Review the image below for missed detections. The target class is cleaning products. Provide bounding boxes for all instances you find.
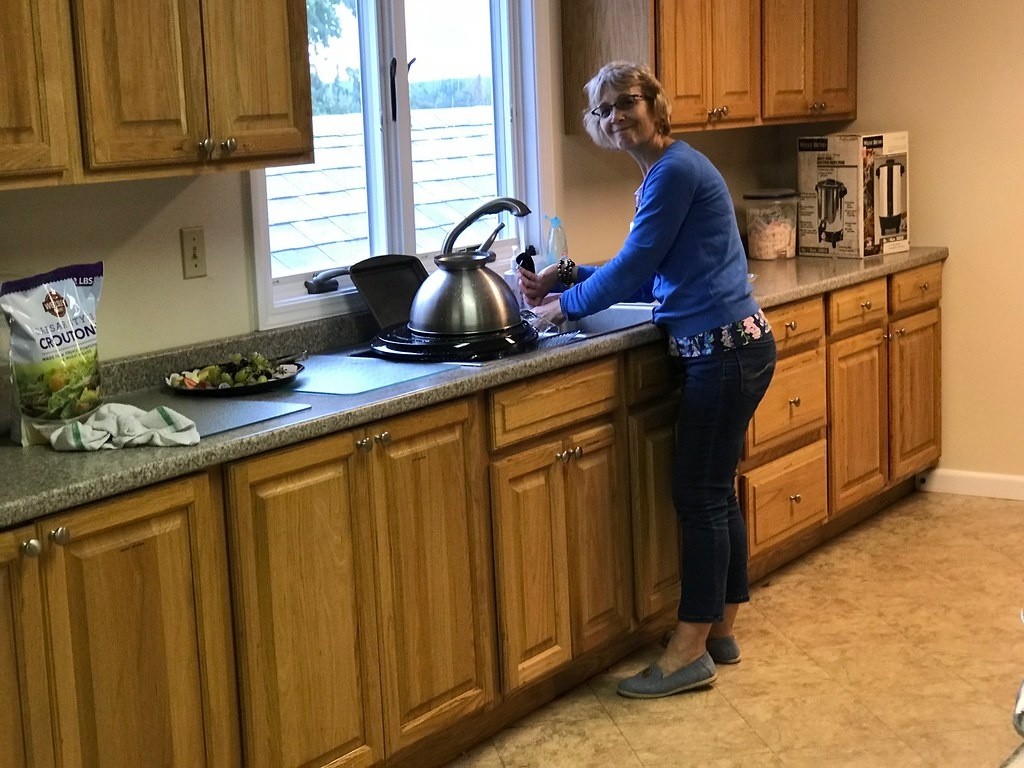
[547,216,570,269]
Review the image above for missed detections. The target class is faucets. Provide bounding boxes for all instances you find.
[443,196,532,254]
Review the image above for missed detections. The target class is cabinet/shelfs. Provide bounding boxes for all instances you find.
[624,338,683,649]
[829,260,942,513]
[560,0,761,136]
[0,0,77,191]
[738,294,831,590]
[482,352,639,732]
[0,464,242,768]
[65,1,316,184]
[213,392,499,768]
[760,0,858,126]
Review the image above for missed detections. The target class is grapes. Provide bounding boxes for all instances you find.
[204,351,275,387]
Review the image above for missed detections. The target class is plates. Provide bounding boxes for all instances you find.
[164,362,305,396]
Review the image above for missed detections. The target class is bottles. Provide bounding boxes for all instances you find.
[545,216,568,265]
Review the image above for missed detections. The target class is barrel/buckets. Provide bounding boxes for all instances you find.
[743,189,800,260]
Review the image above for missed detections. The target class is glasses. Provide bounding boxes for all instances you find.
[591,94,651,118]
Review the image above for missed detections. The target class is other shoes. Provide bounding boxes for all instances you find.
[618,651,719,698]
[663,631,741,663]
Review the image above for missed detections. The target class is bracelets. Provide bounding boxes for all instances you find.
[558,258,576,286]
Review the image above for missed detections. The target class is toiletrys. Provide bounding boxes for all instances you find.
[503,244,521,297]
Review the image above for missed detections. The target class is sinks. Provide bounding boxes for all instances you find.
[517,293,659,338]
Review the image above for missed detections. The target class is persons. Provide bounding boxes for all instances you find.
[518,59,778,700]
